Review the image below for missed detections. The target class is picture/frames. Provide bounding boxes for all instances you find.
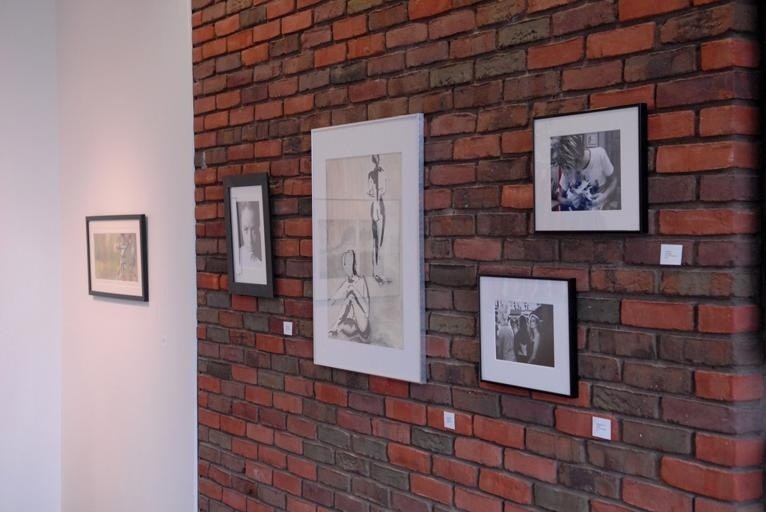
[84,213,148,303]
[533,101,649,236]
[311,111,426,388]
[222,171,274,299]
[481,273,580,398]
